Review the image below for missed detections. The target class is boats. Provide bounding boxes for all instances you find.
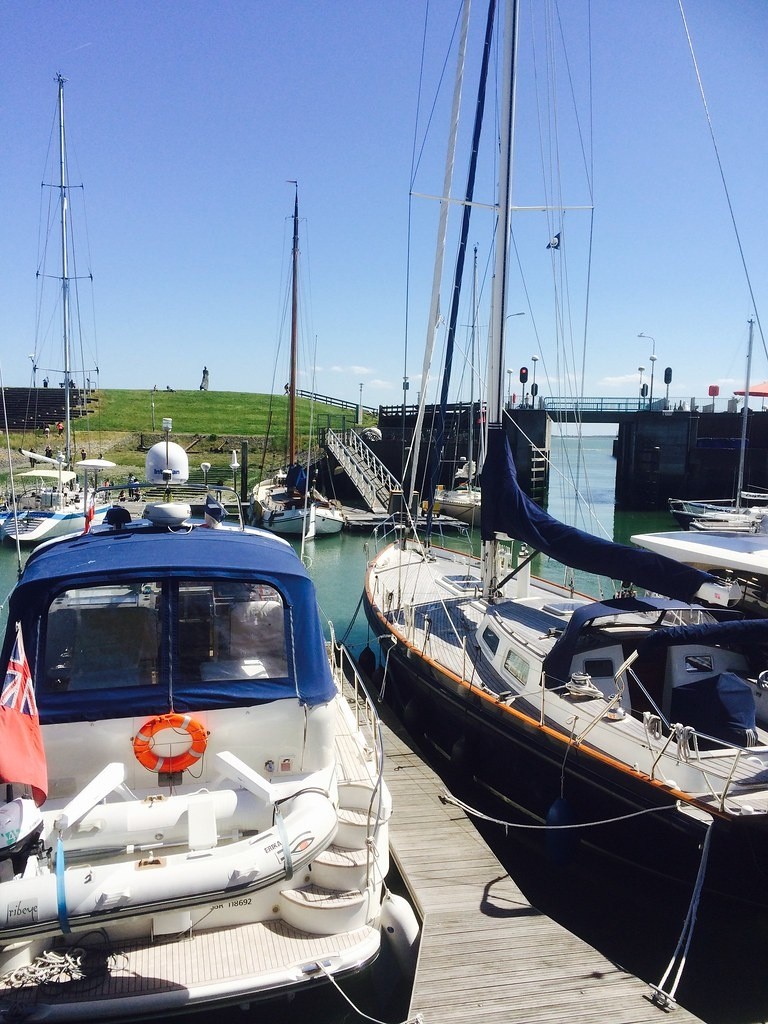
[0,468,423,1024]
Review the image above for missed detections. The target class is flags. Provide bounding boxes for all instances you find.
[81,498,94,535]
[200,495,227,529]
[0,622,47,807]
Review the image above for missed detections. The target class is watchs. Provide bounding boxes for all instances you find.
[57,422,64,435]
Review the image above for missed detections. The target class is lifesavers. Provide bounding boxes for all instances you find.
[132,713,208,773]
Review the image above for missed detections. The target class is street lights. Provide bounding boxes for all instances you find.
[358,383,365,424]
[506,369,514,408]
[635,332,657,411]
[403,377,408,407]
[530,356,539,408]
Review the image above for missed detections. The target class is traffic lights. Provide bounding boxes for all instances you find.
[520,367,527,383]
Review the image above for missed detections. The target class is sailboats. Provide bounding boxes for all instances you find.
[0,70,346,544]
[351,0,767,897]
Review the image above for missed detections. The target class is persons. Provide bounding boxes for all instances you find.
[29,447,35,467]
[45,428,49,438]
[283,383,288,395]
[98,454,104,472]
[31,492,35,497]
[118,490,126,502]
[81,450,87,460]
[127,473,142,502]
[103,479,109,503]
[45,446,53,458]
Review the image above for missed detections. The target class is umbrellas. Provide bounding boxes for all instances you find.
[733,382,768,397]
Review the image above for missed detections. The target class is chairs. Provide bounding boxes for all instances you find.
[202,600,288,681]
[67,607,159,691]
